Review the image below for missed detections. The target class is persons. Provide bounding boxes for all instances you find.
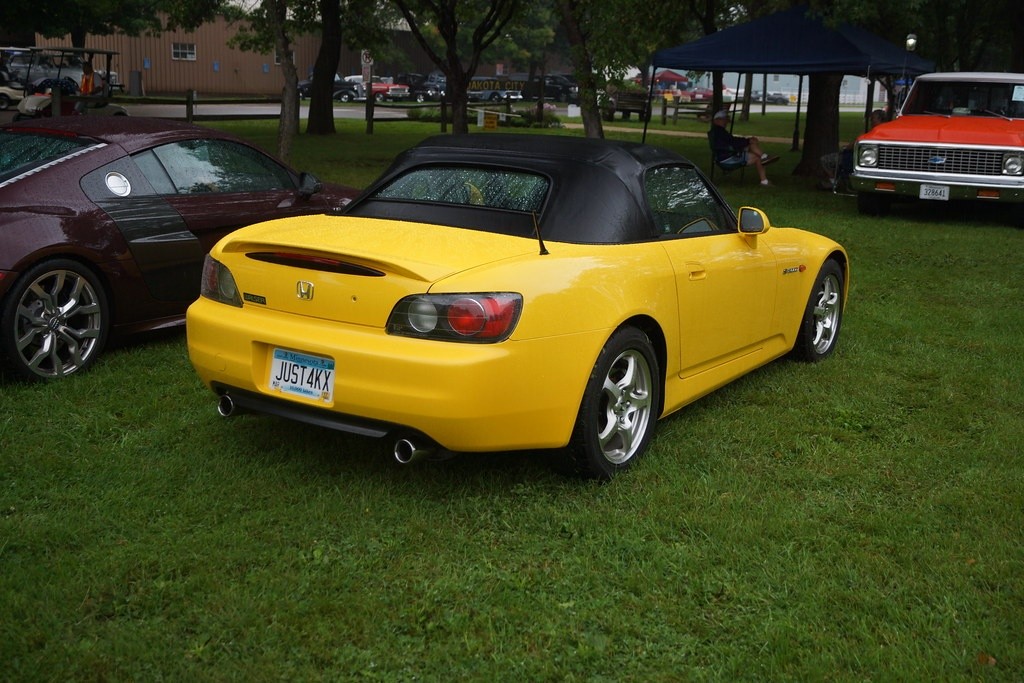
[819,108,885,188]
[710,108,776,187]
[81,61,103,96]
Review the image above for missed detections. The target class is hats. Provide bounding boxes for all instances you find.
[713,110,729,119]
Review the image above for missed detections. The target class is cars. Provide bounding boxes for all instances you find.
[0,114,365,385]
[531,74,580,100]
[0,46,122,111]
[298,72,524,104]
[849,71,1024,218]
[682,87,790,105]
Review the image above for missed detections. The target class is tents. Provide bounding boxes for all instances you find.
[638,6,938,148]
[647,70,688,89]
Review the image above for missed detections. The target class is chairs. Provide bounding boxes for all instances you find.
[707,128,768,188]
[835,140,860,193]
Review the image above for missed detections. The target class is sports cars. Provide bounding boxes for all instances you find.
[186,132,850,478]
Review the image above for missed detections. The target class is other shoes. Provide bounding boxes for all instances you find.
[759,180,776,188]
[761,155,780,166]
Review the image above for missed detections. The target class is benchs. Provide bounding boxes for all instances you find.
[696,102,732,125]
[600,92,656,122]
